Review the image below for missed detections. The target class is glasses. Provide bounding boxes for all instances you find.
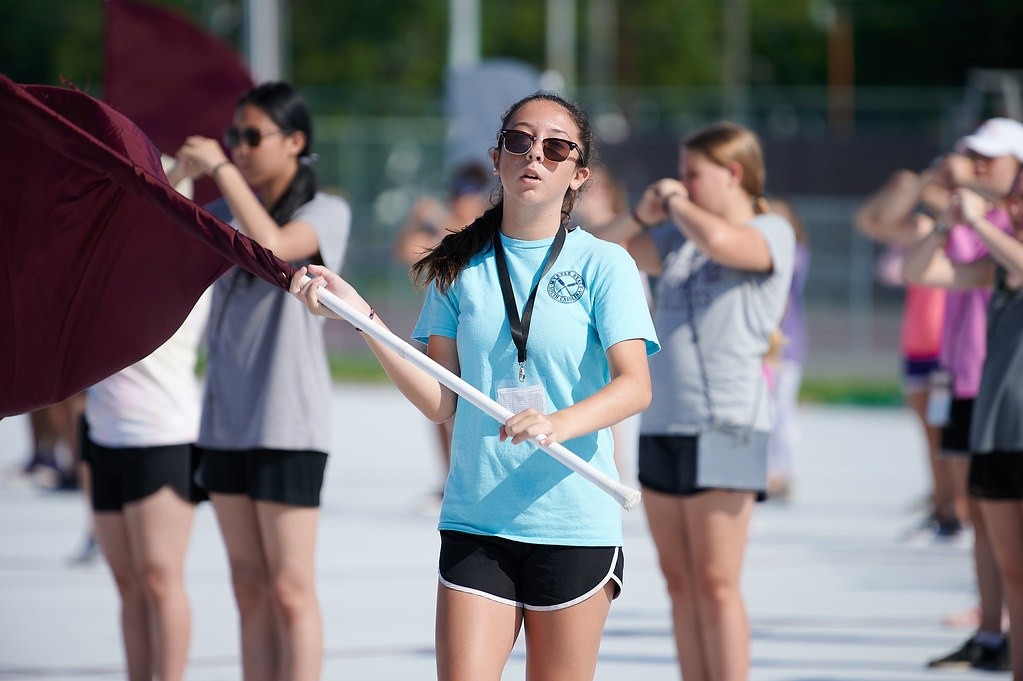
[223,126,290,149]
[495,131,586,167]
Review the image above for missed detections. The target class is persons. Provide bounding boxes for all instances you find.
[289,90,660,680]
[579,164,657,275]
[25,392,87,491]
[165,78,350,680]
[82,153,214,679]
[757,202,811,498]
[597,121,796,678]
[856,120,1022,680]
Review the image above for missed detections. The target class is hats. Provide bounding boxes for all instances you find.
[957,118,1023,162]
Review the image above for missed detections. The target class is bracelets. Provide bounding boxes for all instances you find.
[932,221,951,237]
[354,306,374,331]
[661,191,689,219]
[630,206,655,232]
[211,161,234,182]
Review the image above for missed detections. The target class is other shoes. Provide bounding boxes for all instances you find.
[928,632,1009,676]
[913,513,970,547]
